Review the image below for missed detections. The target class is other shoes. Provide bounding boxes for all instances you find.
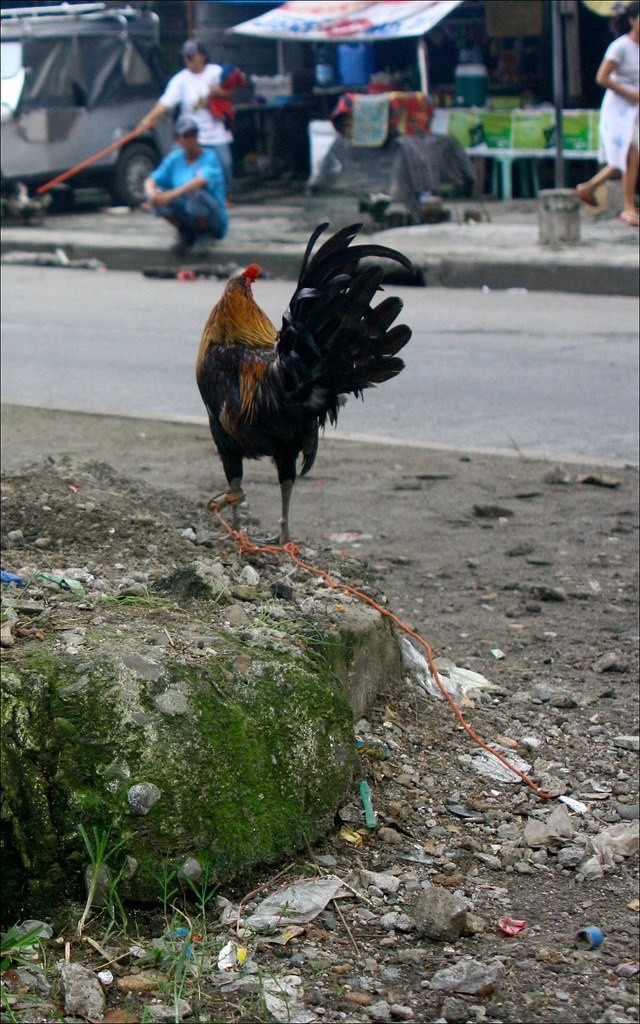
[170,232,196,255]
[576,183,599,206]
[192,236,215,256]
[620,210,640,225]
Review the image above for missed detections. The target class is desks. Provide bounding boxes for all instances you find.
[314,86,369,119]
[228,103,303,178]
[467,145,601,158]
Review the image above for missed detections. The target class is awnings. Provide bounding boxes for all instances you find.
[222,1,468,92]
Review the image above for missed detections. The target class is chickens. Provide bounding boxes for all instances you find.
[194,223,417,526]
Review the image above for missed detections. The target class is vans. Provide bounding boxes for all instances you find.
[1,0,174,208]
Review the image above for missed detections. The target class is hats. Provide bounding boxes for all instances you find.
[180,39,205,57]
[175,117,199,135]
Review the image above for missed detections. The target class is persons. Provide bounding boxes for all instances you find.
[575,2,639,227]
[142,114,228,259]
[136,39,235,203]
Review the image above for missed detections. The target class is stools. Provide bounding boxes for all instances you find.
[494,153,541,199]
